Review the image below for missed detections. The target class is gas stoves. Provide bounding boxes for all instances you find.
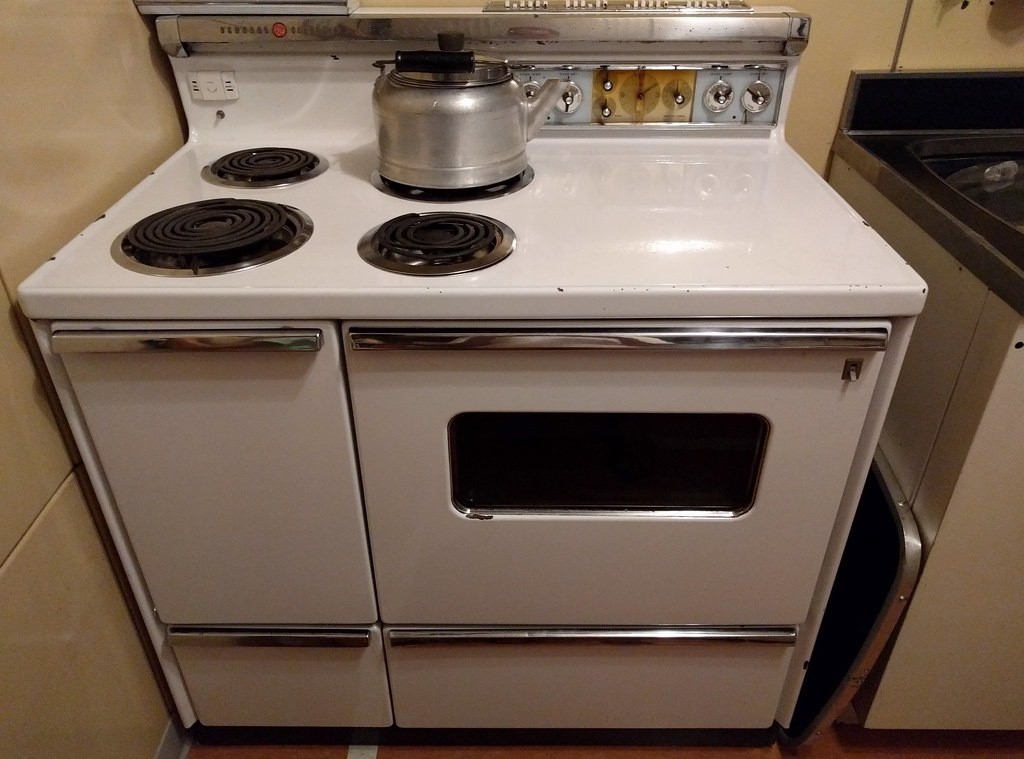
[15,6,929,317]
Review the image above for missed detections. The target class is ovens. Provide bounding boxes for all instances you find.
[30,319,900,730]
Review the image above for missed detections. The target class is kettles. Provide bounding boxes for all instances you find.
[371,32,571,202]
